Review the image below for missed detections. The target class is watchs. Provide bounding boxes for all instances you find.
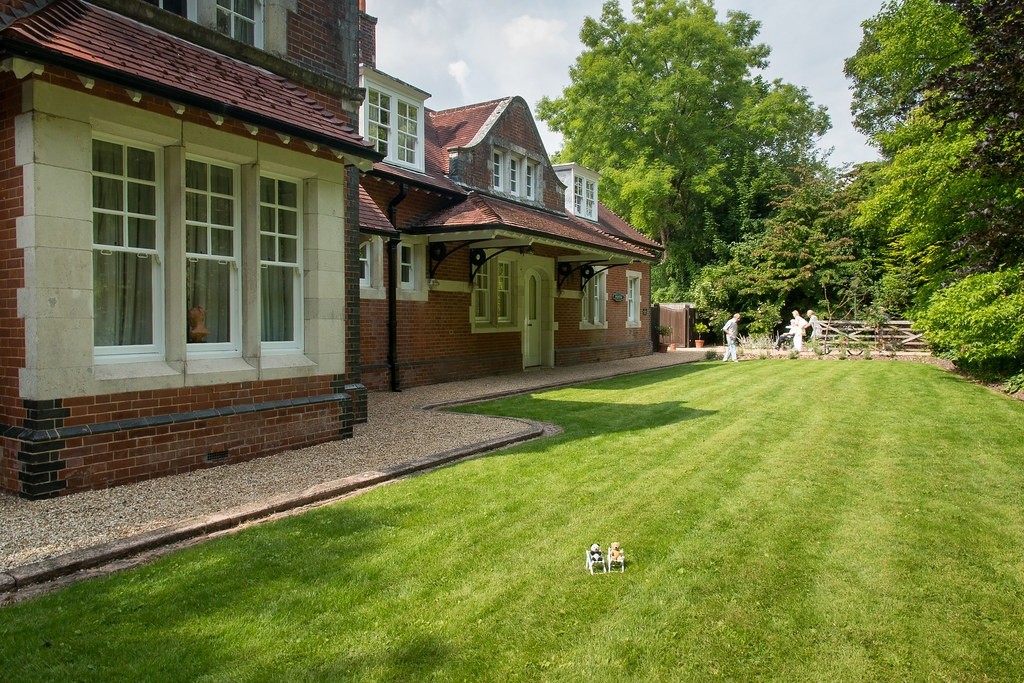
[722,313,741,362]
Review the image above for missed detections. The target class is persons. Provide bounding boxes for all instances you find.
[774,319,795,349]
[804,310,822,351]
[791,310,808,352]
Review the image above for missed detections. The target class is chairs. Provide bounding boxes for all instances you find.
[607,547,625,574]
[585,549,607,575]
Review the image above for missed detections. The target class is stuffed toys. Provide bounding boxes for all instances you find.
[591,542,624,560]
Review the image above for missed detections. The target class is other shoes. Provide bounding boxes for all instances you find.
[734,359,738,362]
[722,358,729,362]
[773,345,780,349]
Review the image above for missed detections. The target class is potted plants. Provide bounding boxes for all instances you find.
[655,324,673,353]
[693,322,710,348]
